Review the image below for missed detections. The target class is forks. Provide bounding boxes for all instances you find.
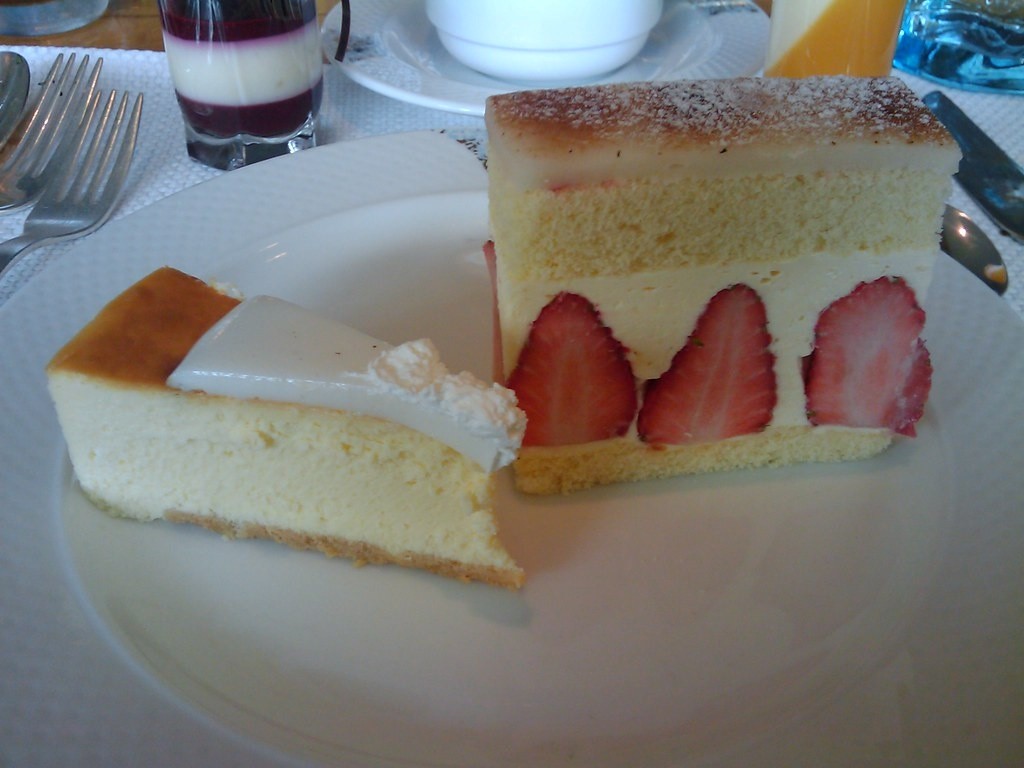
[0,89,143,273]
[0,53,103,218]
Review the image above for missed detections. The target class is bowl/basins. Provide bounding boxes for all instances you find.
[425,0,665,82]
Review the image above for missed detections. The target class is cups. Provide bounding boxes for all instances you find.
[763,0,905,79]
[0,0,110,37]
[894,0,1024,96]
[154,0,323,168]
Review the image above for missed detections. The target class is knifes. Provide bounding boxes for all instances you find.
[919,91,1024,239]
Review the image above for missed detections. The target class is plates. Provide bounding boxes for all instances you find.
[0,132,1024,768]
[316,0,773,117]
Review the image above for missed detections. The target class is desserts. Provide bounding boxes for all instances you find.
[480,79,959,495]
[43,266,526,588]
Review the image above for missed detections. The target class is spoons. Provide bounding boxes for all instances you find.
[938,205,1009,295]
[0,51,31,154]
